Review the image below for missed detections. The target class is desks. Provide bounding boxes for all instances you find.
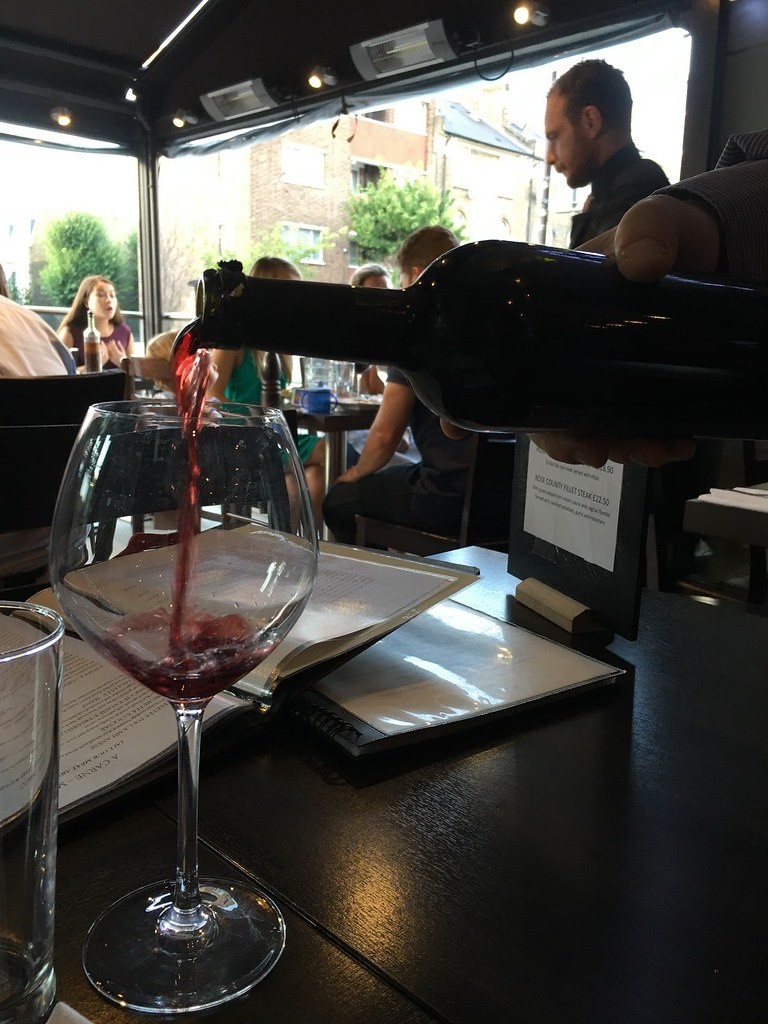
[683,481,768,606]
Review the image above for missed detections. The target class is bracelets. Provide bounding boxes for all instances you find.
[352,465,368,478]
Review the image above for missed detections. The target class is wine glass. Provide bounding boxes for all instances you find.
[48,398,320,1014]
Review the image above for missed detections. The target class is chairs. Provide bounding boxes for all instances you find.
[0,358,132,613]
[0,546,768,1024]
[354,432,515,553]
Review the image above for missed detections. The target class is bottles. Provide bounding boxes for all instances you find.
[82,310,103,374]
[194,242,768,487]
[259,352,282,408]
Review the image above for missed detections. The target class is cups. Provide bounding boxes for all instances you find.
[301,381,338,415]
[0,600,66,1024]
[300,357,356,399]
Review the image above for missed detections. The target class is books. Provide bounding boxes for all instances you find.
[0,526,482,828]
[285,597,629,756]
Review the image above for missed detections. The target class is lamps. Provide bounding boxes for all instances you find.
[513,4,550,28]
[172,108,199,128]
[307,64,338,90]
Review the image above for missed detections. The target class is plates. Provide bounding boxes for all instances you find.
[331,396,383,413]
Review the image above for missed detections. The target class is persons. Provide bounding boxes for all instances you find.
[56,274,136,375]
[202,257,327,536]
[544,60,671,250]
[144,332,182,400]
[322,227,510,547]
[352,264,393,395]
[440,127,768,468]
[0,262,78,379]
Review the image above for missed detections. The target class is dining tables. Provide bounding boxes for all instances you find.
[282,398,379,540]
